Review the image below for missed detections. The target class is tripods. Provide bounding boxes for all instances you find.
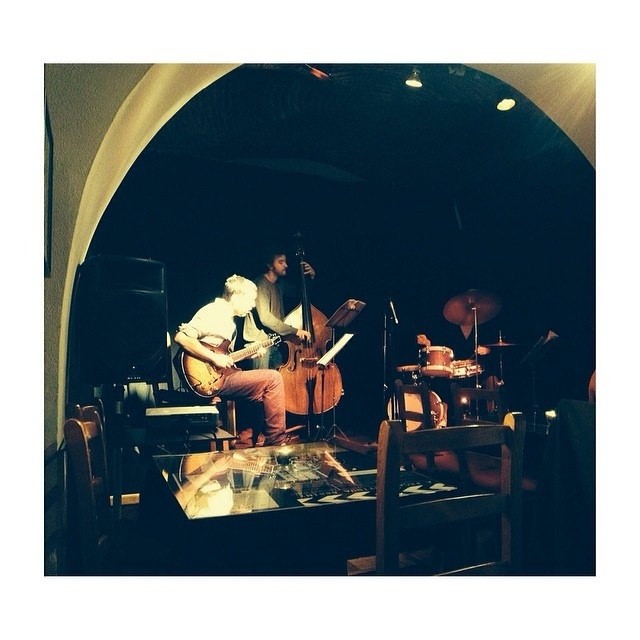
[313,300,365,445]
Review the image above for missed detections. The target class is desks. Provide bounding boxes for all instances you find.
[109,425,236,519]
[153,441,461,575]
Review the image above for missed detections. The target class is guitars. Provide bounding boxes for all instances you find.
[176,333,281,399]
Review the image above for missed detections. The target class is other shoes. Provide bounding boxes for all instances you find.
[234,435,253,449]
[264,433,300,445]
[256,434,266,446]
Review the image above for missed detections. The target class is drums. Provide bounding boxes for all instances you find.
[388,385,443,432]
[418,346,454,377]
[397,365,431,394]
[450,361,483,403]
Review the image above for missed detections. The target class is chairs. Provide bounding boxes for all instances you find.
[64,404,111,576]
[153,333,238,452]
[346,377,537,577]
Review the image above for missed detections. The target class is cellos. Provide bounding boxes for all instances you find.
[278,232,342,439]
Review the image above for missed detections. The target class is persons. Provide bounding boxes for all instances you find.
[174,273,301,446]
[238,247,315,446]
[416,324,492,423]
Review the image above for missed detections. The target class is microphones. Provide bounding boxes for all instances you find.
[389,300,398,324]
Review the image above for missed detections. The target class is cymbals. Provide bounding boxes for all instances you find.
[481,343,520,348]
[443,289,501,326]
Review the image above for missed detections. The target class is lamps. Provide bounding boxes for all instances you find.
[405,65,423,88]
[496,86,516,111]
[305,64,333,80]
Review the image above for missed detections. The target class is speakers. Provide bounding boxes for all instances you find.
[79,256,172,387]
